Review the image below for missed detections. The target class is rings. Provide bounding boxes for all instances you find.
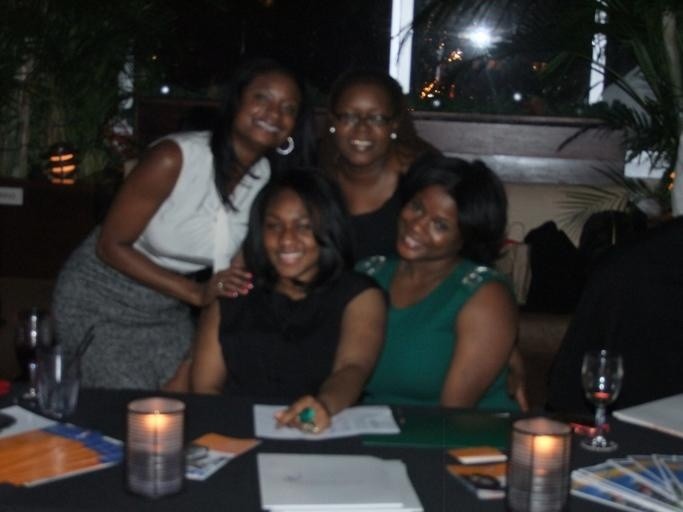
[298,407,313,423]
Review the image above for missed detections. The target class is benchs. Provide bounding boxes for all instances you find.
[491,181,661,408]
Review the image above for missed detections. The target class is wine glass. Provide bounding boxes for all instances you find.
[14,306,55,406]
[580,350,623,453]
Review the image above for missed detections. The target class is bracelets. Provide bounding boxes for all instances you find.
[217,282,224,289]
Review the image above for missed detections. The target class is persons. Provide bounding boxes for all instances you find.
[51,53,303,392]
[192,168,388,434]
[349,158,530,416]
[326,72,515,281]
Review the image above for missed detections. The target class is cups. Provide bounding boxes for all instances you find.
[37,350,79,422]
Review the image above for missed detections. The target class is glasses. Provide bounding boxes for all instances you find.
[340,113,397,127]
